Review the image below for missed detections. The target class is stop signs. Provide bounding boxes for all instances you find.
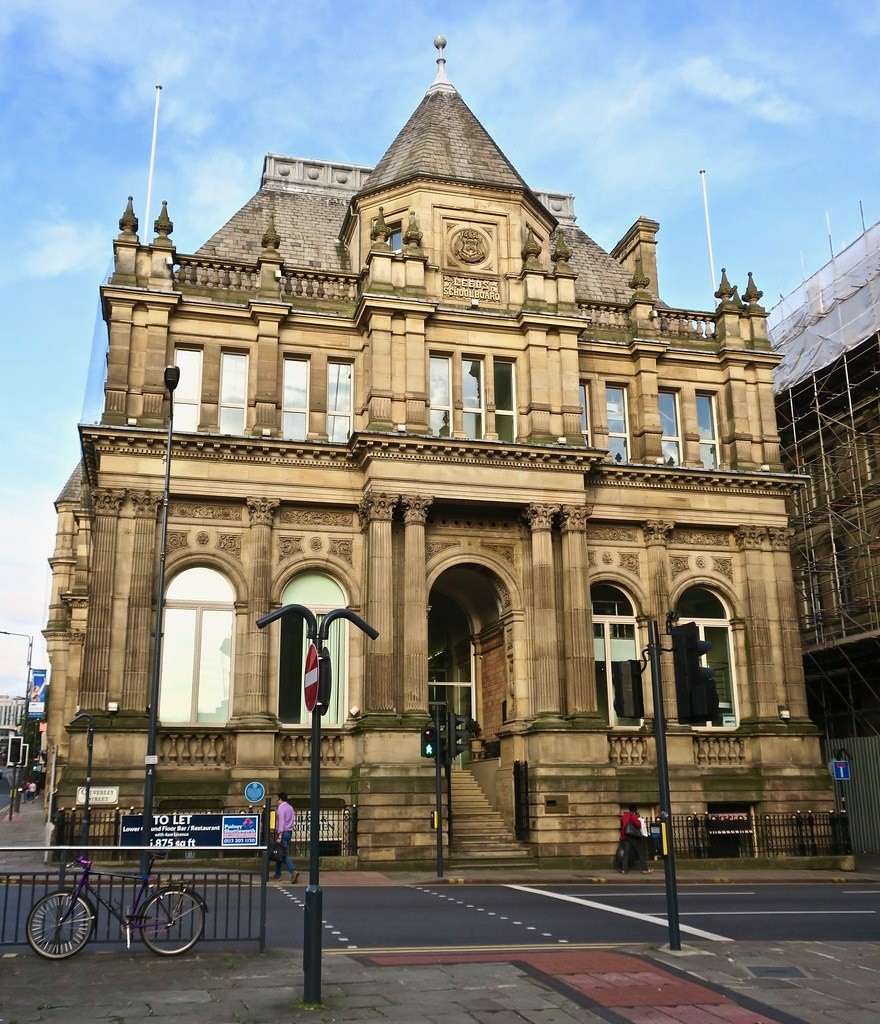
[303,642,320,712]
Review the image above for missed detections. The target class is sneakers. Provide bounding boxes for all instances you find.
[621,869,628,874]
[642,869,654,874]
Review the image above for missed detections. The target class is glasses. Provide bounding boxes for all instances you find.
[277,798,280,801]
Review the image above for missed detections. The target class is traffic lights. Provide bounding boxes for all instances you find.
[671,625,719,723]
[421,726,438,758]
[32,758,39,771]
[449,712,469,759]
[6,736,23,765]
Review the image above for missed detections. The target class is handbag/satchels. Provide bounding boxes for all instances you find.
[616,844,624,865]
[270,843,287,863]
[624,823,642,839]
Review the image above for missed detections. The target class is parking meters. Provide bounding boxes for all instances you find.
[270,811,276,830]
[648,821,667,858]
[431,811,438,829]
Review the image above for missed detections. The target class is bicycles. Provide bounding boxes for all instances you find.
[25,849,210,961]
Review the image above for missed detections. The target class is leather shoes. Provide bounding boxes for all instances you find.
[271,874,281,881]
[291,871,299,885]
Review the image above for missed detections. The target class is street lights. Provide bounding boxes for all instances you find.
[0,632,34,745]
[138,365,181,880]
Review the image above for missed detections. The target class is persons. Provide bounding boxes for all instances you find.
[620,804,655,874]
[20,779,40,804]
[271,793,299,884]
